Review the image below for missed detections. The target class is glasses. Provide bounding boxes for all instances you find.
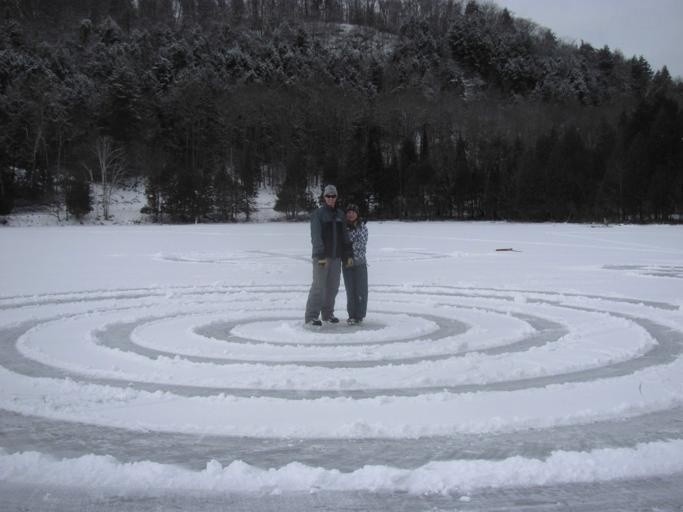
[324,192,337,199]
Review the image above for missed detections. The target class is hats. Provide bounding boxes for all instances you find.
[323,184,338,196]
[345,203,361,215]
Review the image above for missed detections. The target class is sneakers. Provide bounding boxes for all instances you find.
[347,315,365,324]
[303,317,323,327]
[322,313,341,322]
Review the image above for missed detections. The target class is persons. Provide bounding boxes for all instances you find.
[304,184,354,326]
[340,205,368,324]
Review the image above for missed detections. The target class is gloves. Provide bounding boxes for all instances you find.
[343,256,354,268]
[317,255,329,268]
